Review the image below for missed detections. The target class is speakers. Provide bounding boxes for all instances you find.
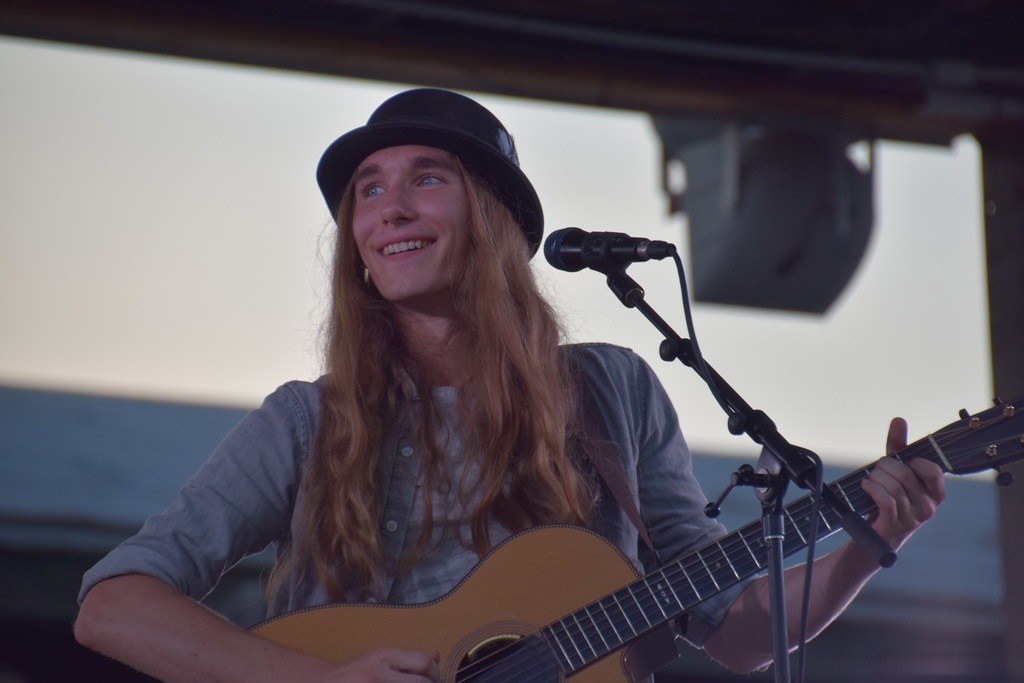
[679,125,872,314]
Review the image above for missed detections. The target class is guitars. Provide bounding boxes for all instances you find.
[247,397,1023,681]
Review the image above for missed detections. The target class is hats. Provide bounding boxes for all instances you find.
[316,87,544,263]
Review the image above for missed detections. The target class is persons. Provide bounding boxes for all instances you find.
[71,89,950,683]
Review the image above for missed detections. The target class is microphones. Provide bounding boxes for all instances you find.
[544,227,676,272]
